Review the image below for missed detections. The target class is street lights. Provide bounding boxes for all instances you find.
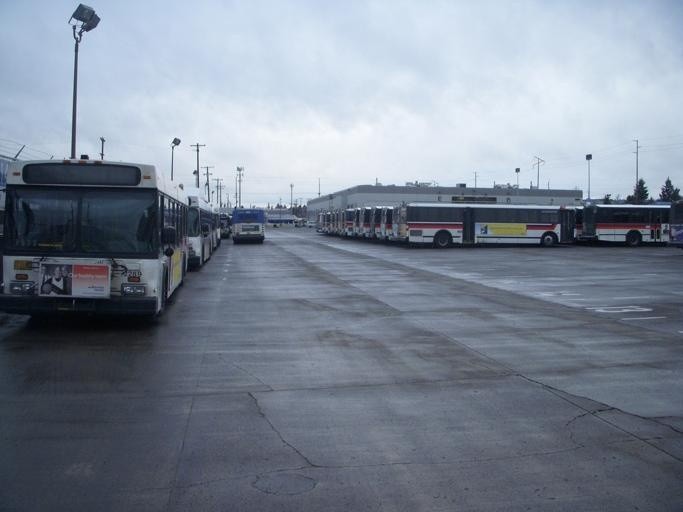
[67,3,100,160]
[170,137,180,180]
[585,154,592,198]
[515,168,519,186]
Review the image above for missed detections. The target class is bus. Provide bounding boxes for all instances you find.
[0,160,231,319]
[267,218,296,228]
[230,207,264,243]
